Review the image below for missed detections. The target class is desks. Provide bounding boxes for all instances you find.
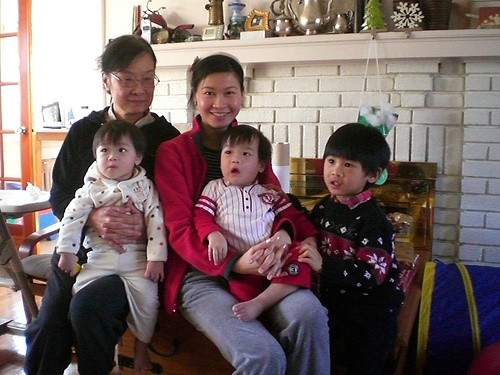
[0,189,52,336]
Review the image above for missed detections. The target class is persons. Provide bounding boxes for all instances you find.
[154,52,330,375]
[193,125,319,321]
[23,35,181,375]
[262,123,404,375]
[56,120,167,373]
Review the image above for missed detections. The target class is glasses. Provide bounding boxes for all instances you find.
[107,73,162,90]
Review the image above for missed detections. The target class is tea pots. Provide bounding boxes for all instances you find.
[288,0,334,36]
[269,0,300,37]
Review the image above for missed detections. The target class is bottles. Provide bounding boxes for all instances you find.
[271,142,290,193]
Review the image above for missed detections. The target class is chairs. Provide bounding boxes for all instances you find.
[19,222,60,325]
[290,158,439,375]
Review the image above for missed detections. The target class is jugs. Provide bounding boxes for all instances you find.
[332,9,354,33]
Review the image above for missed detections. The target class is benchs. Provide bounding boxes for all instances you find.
[118,313,236,375]
